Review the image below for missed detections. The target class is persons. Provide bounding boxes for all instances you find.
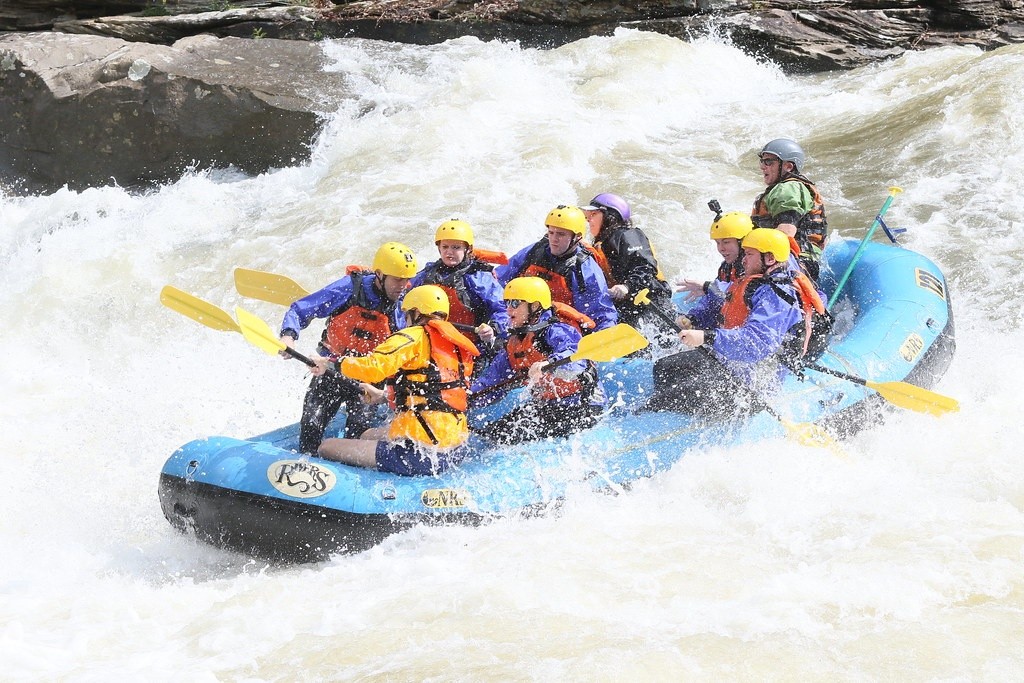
[279,242,417,460]
[307,284,481,477]
[492,205,618,340]
[400,219,509,378]
[466,276,607,444]
[579,192,674,356]
[750,139,828,281]
[636,228,833,417]
[677,212,756,329]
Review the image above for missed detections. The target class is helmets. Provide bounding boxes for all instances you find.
[401,285,449,314]
[741,228,790,262]
[545,205,586,237]
[580,193,630,222]
[504,276,552,310]
[761,138,805,172]
[372,242,417,279]
[435,218,474,245]
[710,211,753,239]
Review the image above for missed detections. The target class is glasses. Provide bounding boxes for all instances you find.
[504,300,519,309]
[760,157,779,166]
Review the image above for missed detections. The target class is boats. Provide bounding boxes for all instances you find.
[156,239,958,571]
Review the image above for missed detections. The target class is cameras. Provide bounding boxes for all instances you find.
[708,200,722,213]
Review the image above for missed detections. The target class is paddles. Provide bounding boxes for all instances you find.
[799,358,960,418]
[634,287,838,446]
[232,265,478,335]
[234,303,369,396]
[828,184,904,311]
[468,324,651,402]
[158,284,243,337]
